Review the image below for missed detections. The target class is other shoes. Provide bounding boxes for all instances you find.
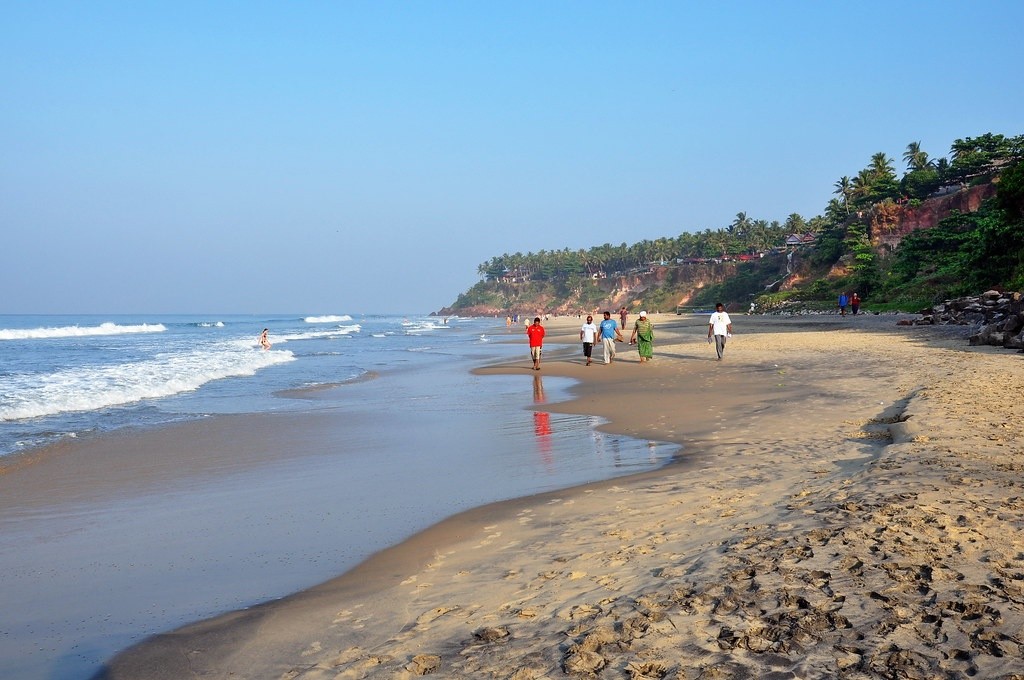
[586,363,590,366]
[536,367,540,370]
[532,367,536,370]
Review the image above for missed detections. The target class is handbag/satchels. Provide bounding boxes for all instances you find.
[848,297,852,306]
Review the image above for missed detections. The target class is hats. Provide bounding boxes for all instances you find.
[639,311,646,317]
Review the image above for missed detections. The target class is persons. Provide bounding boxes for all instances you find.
[838,291,860,317]
[580,316,597,366]
[631,311,655,364]
[597,311,623,365]
[507,314,520,326]
[524,317,531,330]
[620,307,627,330]
[708,303,731,361]
[527,318,545,370]
[258,329,270,350]
